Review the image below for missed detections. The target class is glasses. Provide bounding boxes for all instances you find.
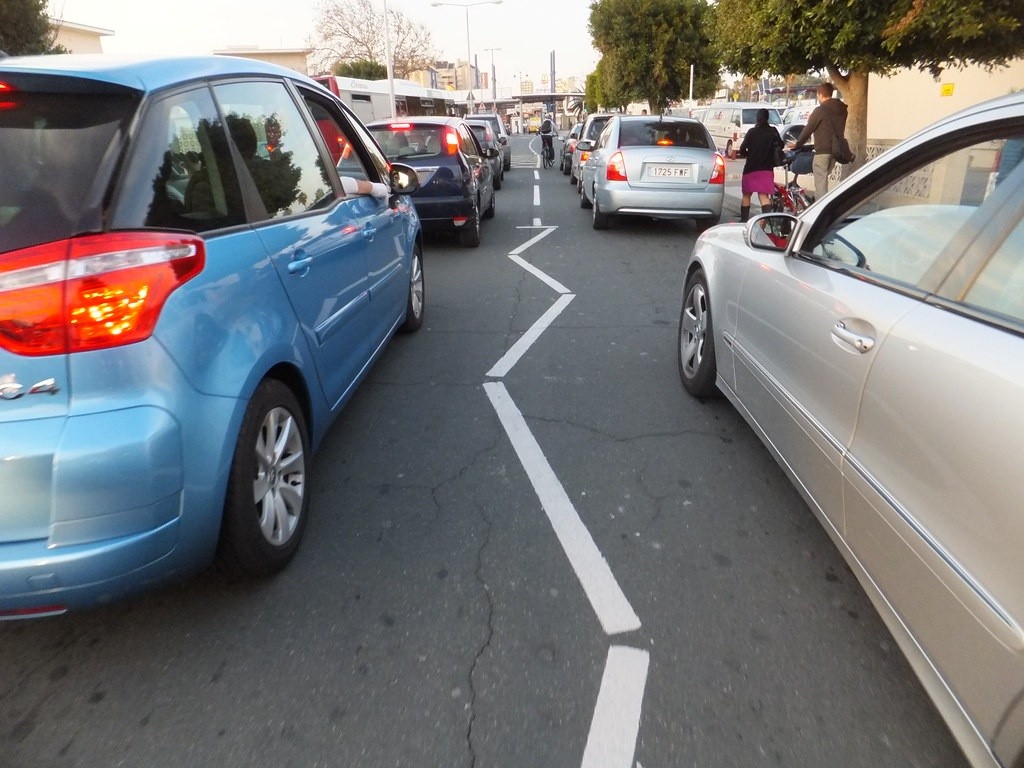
[394,176,400,180]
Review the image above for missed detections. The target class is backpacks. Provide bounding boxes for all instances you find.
[541,119,551,134]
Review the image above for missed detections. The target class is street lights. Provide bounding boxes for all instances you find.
[430,0,504,114]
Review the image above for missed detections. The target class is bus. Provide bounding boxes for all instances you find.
[299,72,460,171]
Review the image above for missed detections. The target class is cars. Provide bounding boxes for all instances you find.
[331,113,514,251]
[559,112,727,233]
[669,86,1024,767]
[1,52,431,633]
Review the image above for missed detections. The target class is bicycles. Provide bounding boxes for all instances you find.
[538,142,555,167]
[766,149,815,216]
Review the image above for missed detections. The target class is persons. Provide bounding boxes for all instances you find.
[739,108,785,224]
[338,176,388,199]
[535,114,560,163]
[641,109,647,116]
[786,82,849,202]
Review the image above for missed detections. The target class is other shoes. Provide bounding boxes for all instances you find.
[541,150,544,155]
[550,160,555,163]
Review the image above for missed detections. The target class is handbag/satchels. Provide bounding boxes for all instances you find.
[771,127,786,166]
[832,135,856,164]
[792,152,813,174]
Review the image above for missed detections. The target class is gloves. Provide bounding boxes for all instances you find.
[369,181,388,206]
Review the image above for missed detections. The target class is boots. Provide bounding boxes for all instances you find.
[761,204,772,213]
[739,204,750,223]
